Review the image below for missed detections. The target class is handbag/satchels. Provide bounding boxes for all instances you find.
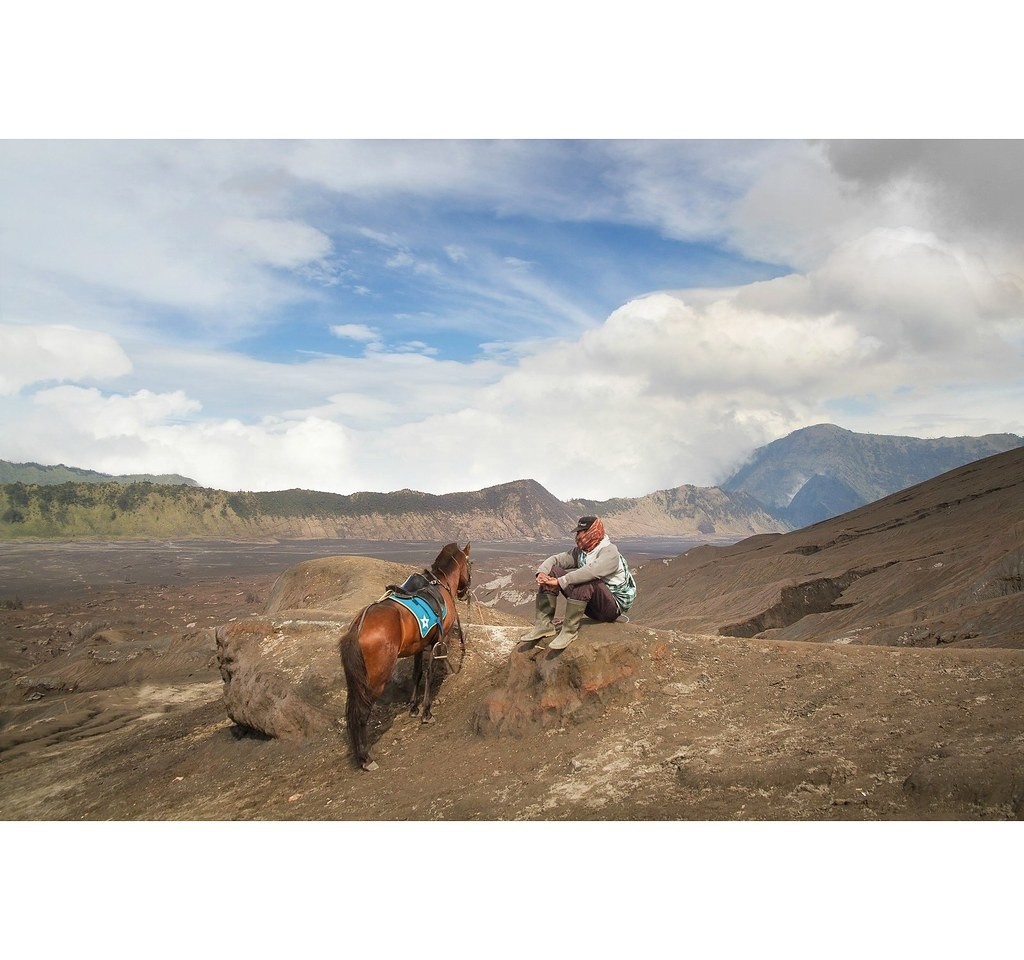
[611,574,637,612]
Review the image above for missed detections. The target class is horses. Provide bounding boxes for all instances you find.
[335,541,473,771]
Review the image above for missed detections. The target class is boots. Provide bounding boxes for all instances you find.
[548,597,587,650]
[520,594,557,641]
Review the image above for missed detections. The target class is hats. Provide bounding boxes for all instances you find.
[571,517,597,532]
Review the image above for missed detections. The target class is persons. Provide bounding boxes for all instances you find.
[519,516,630,650]
